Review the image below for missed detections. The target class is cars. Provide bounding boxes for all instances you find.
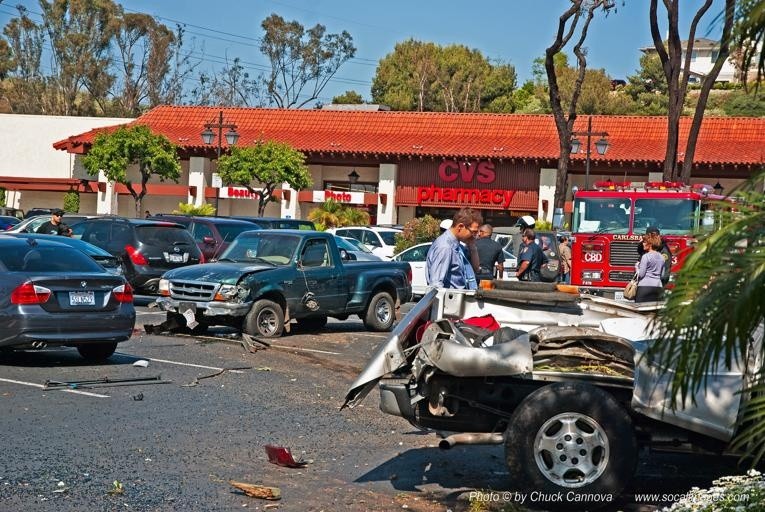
[331,235,383,262]
[54,216,204,301]
[379,280,765,512]
[324,225,404,263]
[0,206,99,238]
[382,242,520,303]
[0,235,137,363]
[146,213,263,264]
[0,233,126,280]
[214,216,317,231]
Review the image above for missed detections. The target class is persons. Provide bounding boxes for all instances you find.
[558,236,572,286]
[634,232,666,303]
[144,210,152,219]
[636,225,674,288]
[515,227,550,281]
[466,224,506,285]
[514,213,538,281]
[424,207,485,290]
[35,208,75,240]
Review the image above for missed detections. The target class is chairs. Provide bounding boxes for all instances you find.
[268,238,326,266]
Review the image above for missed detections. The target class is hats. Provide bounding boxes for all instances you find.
[646,226,659,234]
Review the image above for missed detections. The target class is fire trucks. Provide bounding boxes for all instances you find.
[553,182,765,302]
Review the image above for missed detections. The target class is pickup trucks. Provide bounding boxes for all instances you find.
[156,229,412,340]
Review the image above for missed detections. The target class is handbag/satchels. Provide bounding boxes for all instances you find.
[623,279,638,300]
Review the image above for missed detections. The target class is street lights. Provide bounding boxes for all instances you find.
[199,111,241,217]
[570,116,612,191]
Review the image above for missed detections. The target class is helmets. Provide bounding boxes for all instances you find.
[515,215,535,234]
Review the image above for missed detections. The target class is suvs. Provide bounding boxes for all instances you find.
[474,227,562,286]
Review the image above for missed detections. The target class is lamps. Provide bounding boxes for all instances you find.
[714,183,724,195]
[347,169,360,183]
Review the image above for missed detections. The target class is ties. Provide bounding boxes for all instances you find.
[457,247,478,290]
